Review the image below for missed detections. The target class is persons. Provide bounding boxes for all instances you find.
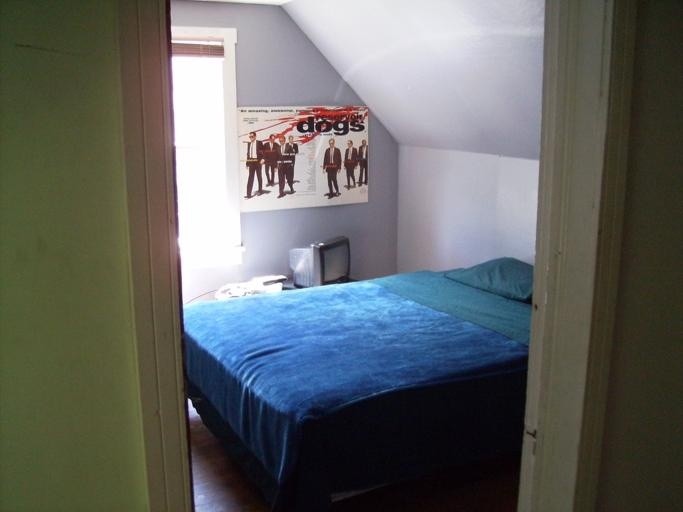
[263,133,278,187]
[355,138,370,187]
[321,138,342,199]
[273,134,296,199]
[344,139,359,190]
[242,130,263,199]
[283,134,299,182]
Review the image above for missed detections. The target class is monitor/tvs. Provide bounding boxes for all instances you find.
[290,236,350,287]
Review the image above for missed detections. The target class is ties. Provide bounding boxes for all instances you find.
[331,148,333,163]
[251,143,253,159]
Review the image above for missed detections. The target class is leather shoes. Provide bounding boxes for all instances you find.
[328,183,368,199]
[244,183,295,198]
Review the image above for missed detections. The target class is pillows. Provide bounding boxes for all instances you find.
[445,257,534,305]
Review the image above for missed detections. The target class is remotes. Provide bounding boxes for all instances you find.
[263,275,287,284]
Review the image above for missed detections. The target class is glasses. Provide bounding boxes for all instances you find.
[249,135,253,138]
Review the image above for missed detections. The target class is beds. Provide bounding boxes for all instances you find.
[183,269,532,511]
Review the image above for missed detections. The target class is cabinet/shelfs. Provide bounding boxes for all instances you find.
[263,273,359,292]
